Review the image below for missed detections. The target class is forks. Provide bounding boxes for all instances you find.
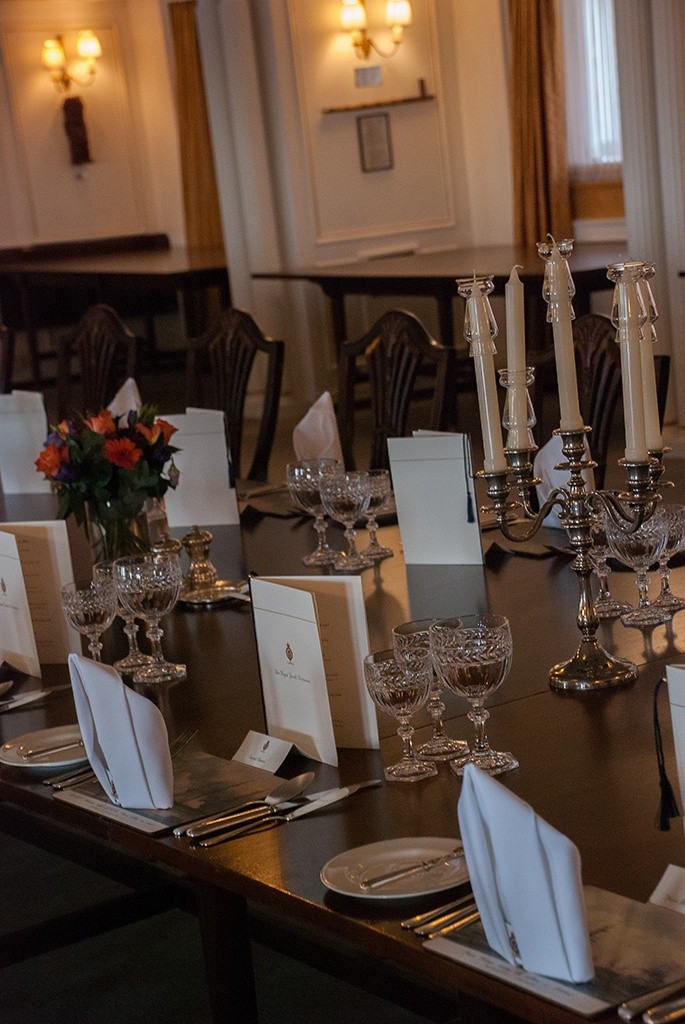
[169,728,197,757]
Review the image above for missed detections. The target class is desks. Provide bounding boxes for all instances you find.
[2,476,685,1024]
[250,244,630,433]
[0,247,228,393]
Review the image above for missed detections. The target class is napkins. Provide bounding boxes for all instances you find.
[533,435,595,529]
[457,762,596,983]
[292,391,345,476]
[101,377,141,417]
[67,653,174,811]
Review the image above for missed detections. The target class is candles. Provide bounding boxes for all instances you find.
[468,250,664,472]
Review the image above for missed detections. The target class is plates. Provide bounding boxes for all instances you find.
[0,724,88,768]
[321,837,470,899]
[179,579,250,608]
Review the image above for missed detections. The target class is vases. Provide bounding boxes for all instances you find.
[86,500,152,565]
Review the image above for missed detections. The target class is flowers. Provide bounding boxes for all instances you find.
[34,402,185,559]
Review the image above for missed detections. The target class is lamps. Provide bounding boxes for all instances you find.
[327,0,413,62]
[40,29,102,93]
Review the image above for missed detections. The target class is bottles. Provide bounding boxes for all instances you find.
[182,526,218,585]
[151,533,186,585]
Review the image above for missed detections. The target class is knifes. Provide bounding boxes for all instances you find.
[200,784,361,846]
[0,684,72,712]
[187,779,382,837]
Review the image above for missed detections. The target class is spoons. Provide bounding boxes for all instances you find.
[0,681,13,695]
[173,772,314,836]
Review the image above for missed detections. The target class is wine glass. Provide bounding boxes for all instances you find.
[563,491,634,617]
[649,506,685,612]
[360,470,393,558]
[93,560,154,673]
[604,506,671,625]
[393,619,470,760]
[61,579,118,663]
[318,471,374,569]
[286,457,346,566]
[113,553,186,683]
[364,647,438,782]
[429,615,520,776]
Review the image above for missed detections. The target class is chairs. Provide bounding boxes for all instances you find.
[0,296,621,540]
